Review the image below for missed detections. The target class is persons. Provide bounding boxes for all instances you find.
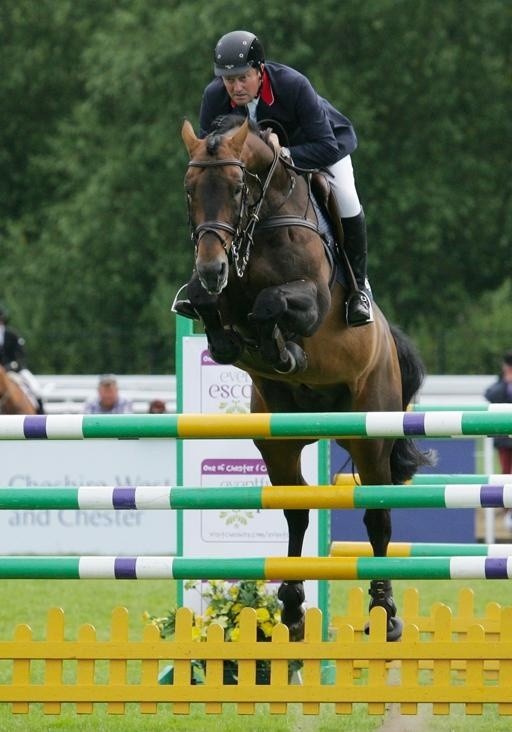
[172,30,376,327]
[81,375,137,415]
[481,354,511,529]
[149,401,167,414]
[0,307,45,415]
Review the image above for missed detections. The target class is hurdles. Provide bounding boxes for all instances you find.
[0,410,512,685]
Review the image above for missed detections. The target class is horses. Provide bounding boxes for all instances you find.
[181,116,439,642]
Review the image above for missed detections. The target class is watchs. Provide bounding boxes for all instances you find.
[280,145,289,160]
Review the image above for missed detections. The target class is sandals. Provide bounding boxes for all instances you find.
[341,204,372,322]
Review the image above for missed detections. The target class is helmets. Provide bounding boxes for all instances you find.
[212,30,265,77]
